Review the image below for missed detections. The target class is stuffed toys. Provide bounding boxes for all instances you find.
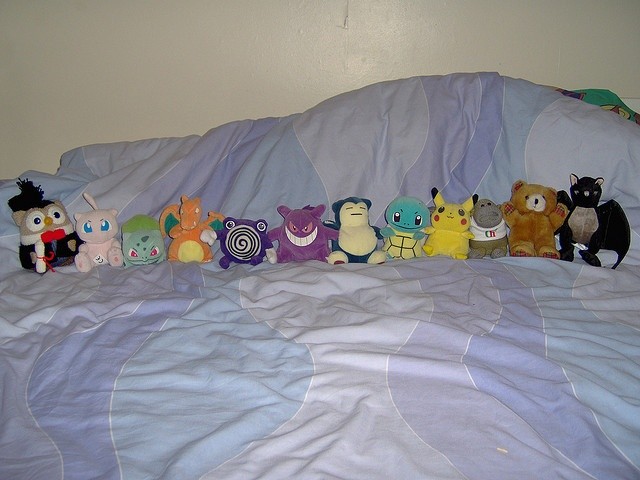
[322,196,386,264]
[553,173,630,269]
[7,176,82,273]
[159,195,225,262]
[379,196,429,259]
[268,203,337,263]
[421,186,478,259]
[501,179,567,259]
[199,216,276,268]
[73,192,123,272]
[468,197,507,259]
[122,214,167,266]
